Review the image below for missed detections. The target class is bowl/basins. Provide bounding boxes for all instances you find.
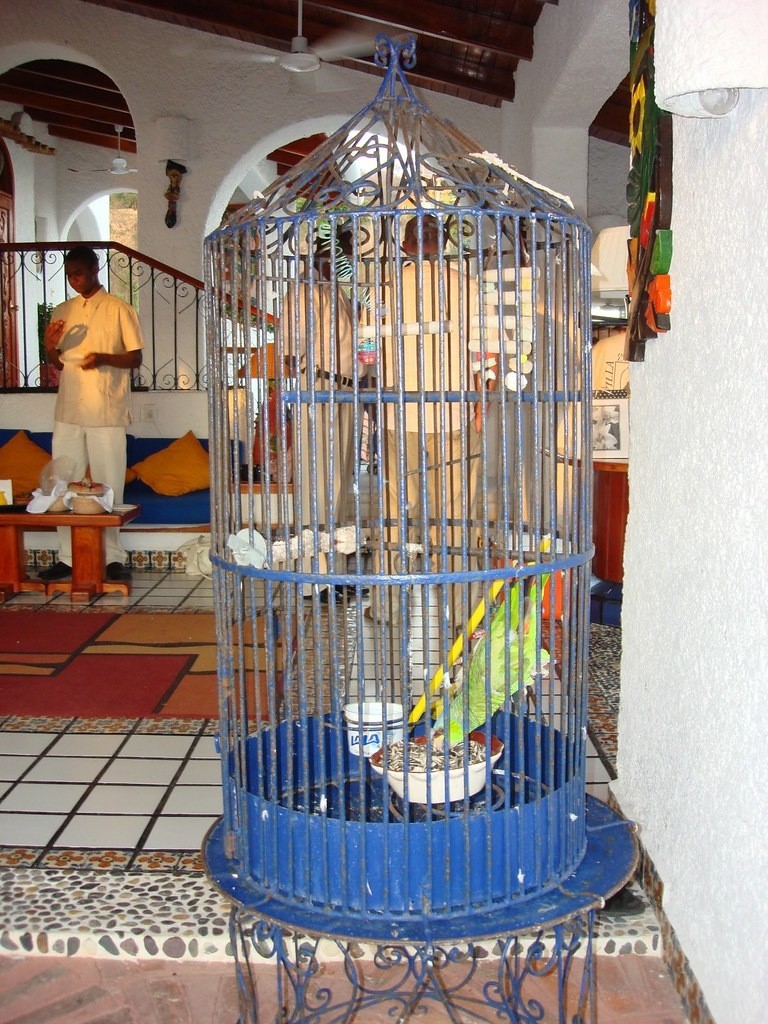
[367,730,504,803]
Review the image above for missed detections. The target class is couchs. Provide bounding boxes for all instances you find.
[0,428,243,525]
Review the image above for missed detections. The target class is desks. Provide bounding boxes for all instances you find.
[538,446,629,583]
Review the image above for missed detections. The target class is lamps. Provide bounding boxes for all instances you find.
[237,344,297,380]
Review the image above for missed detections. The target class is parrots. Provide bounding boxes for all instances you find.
[415,572,559,753]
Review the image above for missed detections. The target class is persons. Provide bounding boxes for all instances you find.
[37,248,145,584]
[275,213,509,628]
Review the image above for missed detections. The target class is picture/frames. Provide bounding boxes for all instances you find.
[577,398,629,459]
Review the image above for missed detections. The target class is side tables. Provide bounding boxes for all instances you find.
[229,483,293,532]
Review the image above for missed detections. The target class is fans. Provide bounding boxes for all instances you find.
[591,298,625,311]
[190,0,419,73]
[66,124,139,174]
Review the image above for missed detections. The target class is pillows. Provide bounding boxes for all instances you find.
[86,464,137,484]
[0,431,52,497]
[130,429,233,497]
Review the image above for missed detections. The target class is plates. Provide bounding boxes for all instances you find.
[59,354,88,366]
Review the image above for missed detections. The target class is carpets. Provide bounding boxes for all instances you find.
[0,608,308,723]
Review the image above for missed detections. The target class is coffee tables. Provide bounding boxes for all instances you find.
[0,504,141,605]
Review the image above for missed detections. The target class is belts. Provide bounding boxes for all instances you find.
[301,367,362,388]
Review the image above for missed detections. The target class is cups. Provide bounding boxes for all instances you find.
[344,704,404,757]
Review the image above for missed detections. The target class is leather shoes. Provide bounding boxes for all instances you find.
[336,584,369,597]
[303,585,350,603]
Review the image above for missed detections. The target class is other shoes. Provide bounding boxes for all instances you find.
[106,562,132,580]
[39,561,72,580]
